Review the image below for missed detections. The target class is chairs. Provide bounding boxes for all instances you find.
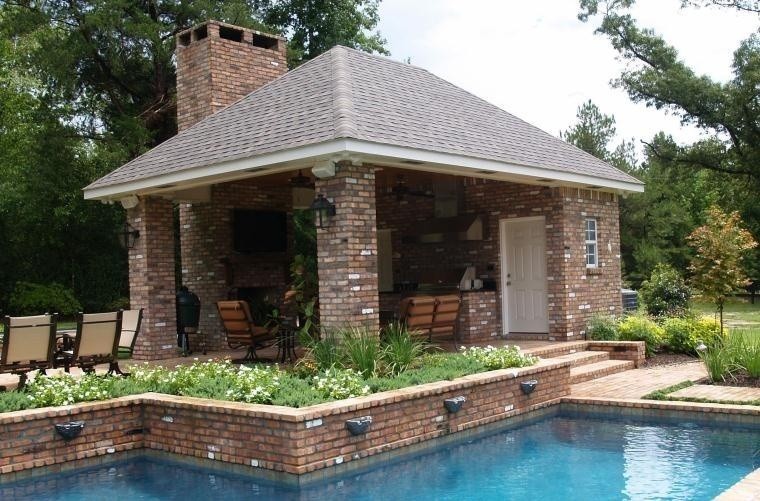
[398,294,437,345]
[213,299,276,365]
[0,307,145,392]
[429,294,462,353]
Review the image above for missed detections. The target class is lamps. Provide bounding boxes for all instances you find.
[309,193,336,232]
[346,415,372,436]
[520,380,538,395]
[116,219,141,249]
[55,420,86,442]
[444,396,466,412]
[291,184,313,210]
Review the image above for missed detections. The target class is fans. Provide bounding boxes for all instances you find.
[256,169,316,191]
[375,174,437,199]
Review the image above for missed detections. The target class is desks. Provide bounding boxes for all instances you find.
[266,311,305,363]
